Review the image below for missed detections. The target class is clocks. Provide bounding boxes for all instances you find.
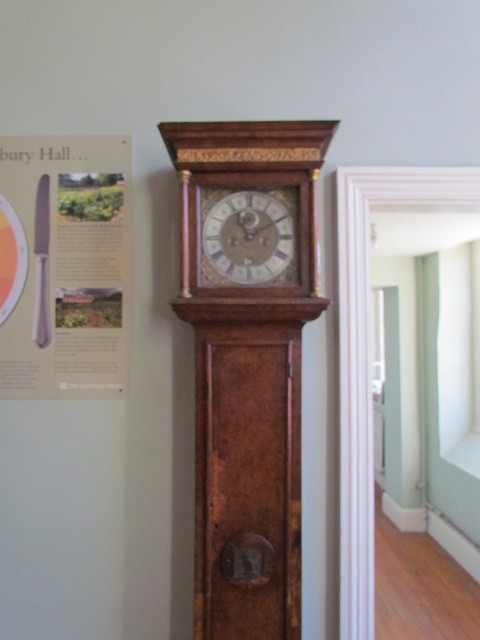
[197,184,300,286]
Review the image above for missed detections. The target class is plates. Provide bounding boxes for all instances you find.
[0,194,28,326]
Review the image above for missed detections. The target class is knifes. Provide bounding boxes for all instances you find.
[34,175,52,347]
